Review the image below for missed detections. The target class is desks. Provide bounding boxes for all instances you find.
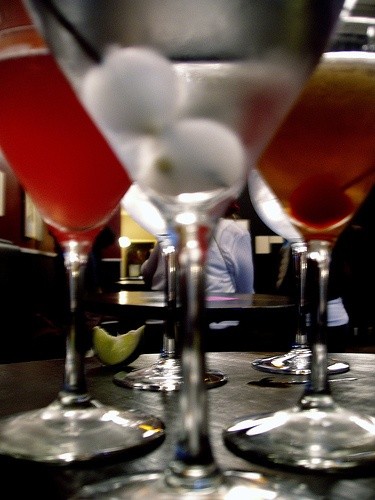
[0,288,375,500]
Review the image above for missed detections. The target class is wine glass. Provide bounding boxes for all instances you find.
[1,0,375,500]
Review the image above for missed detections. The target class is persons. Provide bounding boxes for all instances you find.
[140,217,256,293]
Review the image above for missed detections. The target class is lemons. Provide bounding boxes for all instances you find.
[91,324,148,369]
